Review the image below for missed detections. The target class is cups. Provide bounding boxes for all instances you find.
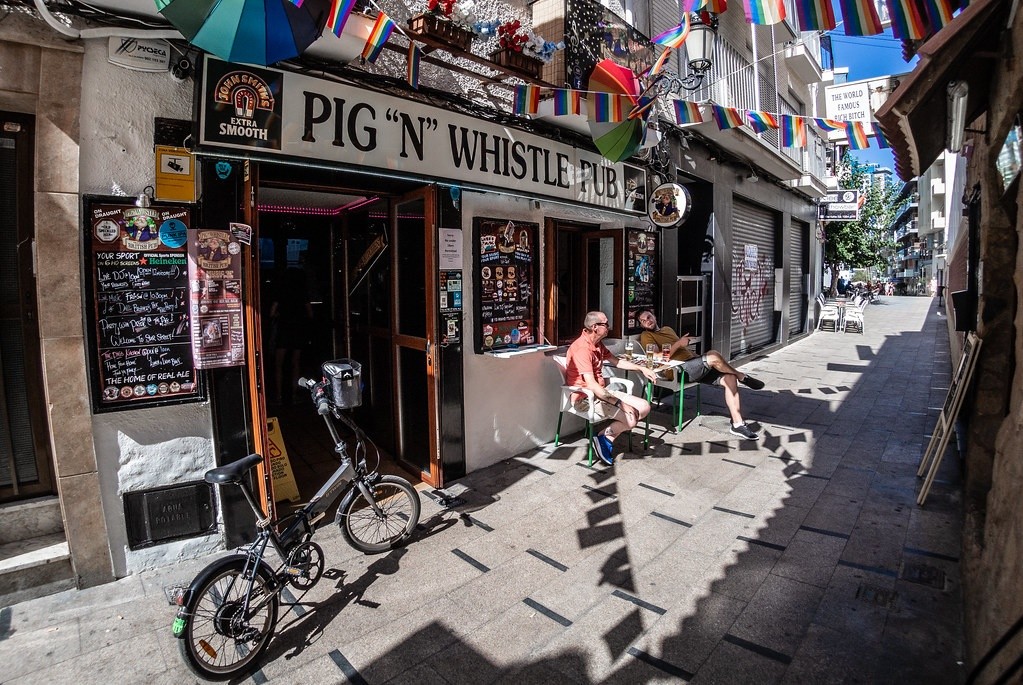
[662,344,671,363]
[624,341,634,361]
[646,344,654,370]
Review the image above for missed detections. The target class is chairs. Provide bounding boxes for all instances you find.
[815,291,869,336]
[634,339,701,432]
[551,354,635,468]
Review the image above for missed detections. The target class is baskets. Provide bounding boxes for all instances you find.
[322,358,363,407]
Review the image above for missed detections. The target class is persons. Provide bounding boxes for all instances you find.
[635,306,765,440]
[837,275,855,294]
[866,280,871,290]
[884,282,890,296]
[888,282,895,296]
[567,311,650,465]
[876,280,882,288]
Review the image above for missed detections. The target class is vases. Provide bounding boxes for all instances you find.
[407,12,478,58]
[488,47,545,84]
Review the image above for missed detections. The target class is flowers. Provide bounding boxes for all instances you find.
[425,0,503,44]
[494,20,566,63]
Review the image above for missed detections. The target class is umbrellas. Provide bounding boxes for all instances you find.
[585,59,647,164]
[152,0,334,68]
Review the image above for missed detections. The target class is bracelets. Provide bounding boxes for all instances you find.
[615,399,622,407]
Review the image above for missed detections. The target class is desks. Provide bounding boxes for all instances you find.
[826,301,855,327]
[825,303,859,334]
[603,353,686,450]
[825,297,851,301]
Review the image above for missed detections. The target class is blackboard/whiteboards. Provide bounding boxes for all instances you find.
[82,194,208,415]
[472,217,540,354]
[624,226,663,336]
[940,331,983,435]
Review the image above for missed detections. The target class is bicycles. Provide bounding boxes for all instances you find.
[172,358,421,682]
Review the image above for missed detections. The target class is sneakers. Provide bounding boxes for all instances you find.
[730,422,759,440]
[591,432,600,458]
[738,374,764,390]
[593,434,614,465]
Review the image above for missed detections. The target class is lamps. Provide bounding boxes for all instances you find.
[945,79,969,154]
[651,5,719,100]
[135,185,155,207]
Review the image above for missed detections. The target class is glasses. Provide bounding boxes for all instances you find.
[594,322,608,326]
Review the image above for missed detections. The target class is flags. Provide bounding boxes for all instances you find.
[288,0,304,8]
[512,84,891,151]
[361,11,396,65]
[325,0,356,39]
[407,41,421,90]
[649,0,953,76]
